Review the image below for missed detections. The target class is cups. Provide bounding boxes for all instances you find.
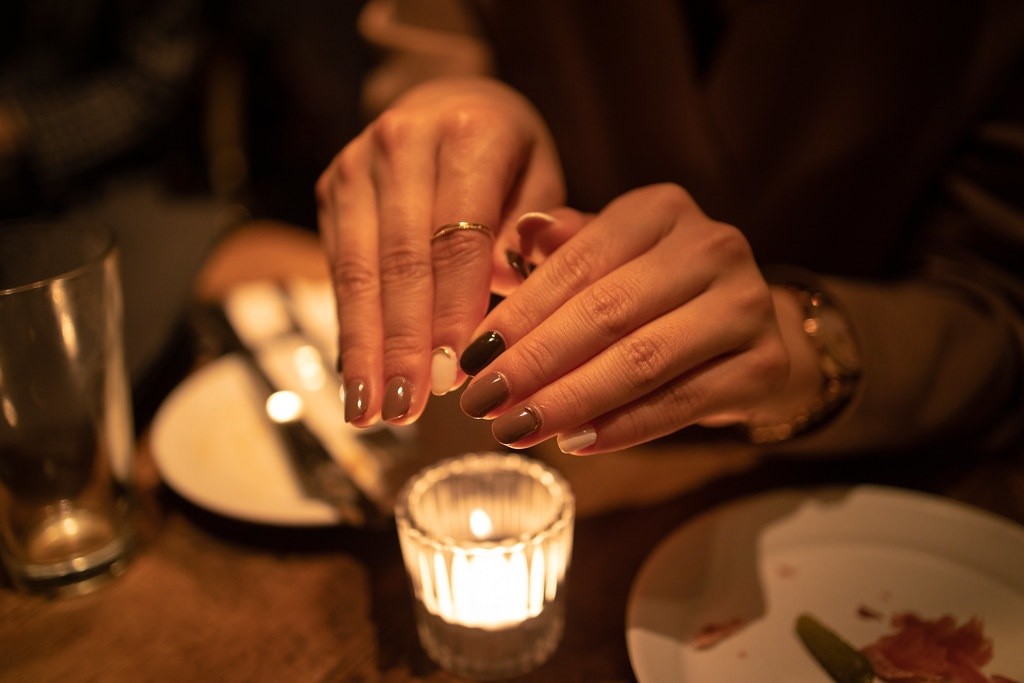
[0,217,140,602]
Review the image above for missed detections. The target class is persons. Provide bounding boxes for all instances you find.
[316,0,1024,456]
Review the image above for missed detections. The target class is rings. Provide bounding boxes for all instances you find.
[430,221,495,244]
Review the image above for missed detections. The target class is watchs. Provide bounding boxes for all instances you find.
[747,279,861,445]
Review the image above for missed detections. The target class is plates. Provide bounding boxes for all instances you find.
[624,479,1024,682]
[149,344,757,528]
